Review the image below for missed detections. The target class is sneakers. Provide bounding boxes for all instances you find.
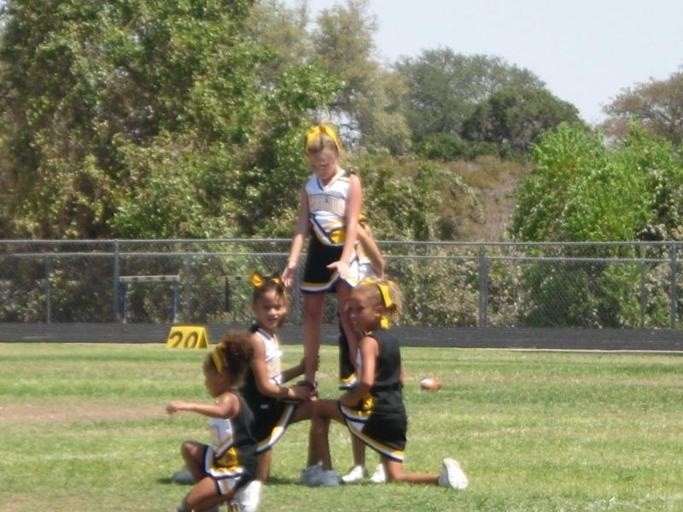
[236,480,261,512]
[172,468,195,484]
[284,381,319,405]
[341,466,368,483]
[370,463,387,483]
[441,457,469,490]
[300,464,338,486]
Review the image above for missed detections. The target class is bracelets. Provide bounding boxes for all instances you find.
[287,257,298,263]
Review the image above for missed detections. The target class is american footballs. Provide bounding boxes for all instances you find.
[420,378,442,390]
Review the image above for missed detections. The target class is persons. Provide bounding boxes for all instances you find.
[301,275,469,489]
[334,214,386,485]
[175,274,323,480]
[280,123,363,389]
[167,326,262,512]
